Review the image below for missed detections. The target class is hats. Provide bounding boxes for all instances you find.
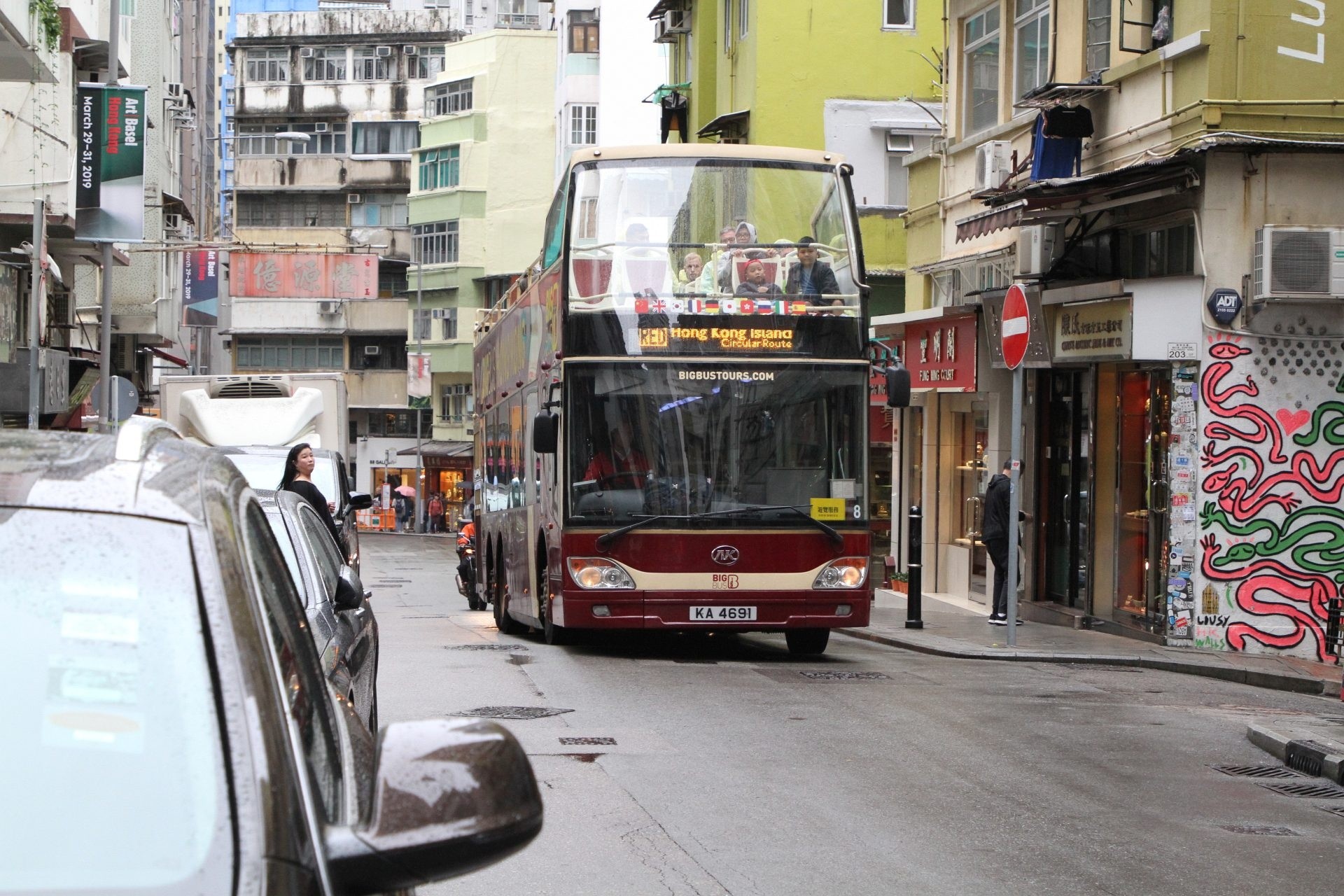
[744,258,763,280]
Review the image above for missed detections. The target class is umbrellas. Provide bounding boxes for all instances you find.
[393,485,416,497]
[455,481,474,489]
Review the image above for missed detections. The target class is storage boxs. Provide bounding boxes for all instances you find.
[655,21,672,43]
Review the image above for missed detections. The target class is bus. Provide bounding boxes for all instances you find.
[468,148,908,657]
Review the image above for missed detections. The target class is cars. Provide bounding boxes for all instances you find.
[158,442,377,763]
[2,409,549,896]
[160,372,350,478]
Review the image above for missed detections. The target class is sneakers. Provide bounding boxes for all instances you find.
[988,613,1024,625]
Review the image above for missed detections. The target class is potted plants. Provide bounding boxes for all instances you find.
[889,572,908,594]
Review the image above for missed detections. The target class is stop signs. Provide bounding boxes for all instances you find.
[996,285,1032,368]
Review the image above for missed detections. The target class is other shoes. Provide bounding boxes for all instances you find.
[462,582,468,588]
[399,531,404,533]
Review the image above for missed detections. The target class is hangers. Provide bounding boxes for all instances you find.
[1039,95,1080,110]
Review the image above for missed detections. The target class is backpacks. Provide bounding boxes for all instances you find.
[396,498,403,513]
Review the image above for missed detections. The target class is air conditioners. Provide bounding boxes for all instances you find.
[315,122,329,133]
[664,10,691,33]
[299,48,315,58]
[446,387,456,395]
[1019,220,1064,283]
[47,292,75,328]
[376,46,393,57]
[973,140,1017,196]
[166,82,191,119]
[365,345,382,355]
[317,302,337,314]
[403,45,420,56]
[348,193,361,203]
[163,213,194,238]
[433,309,449,319]
[116,334,137,373]
[458,385,470,395]
[1252,224,1344,307]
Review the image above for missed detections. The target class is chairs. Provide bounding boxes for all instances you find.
[569,245,672,310]
[732,246,834,294]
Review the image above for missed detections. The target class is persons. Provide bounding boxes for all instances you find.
[980,456,1034,625]
[701,221,795,299]
[785,236,845,316]
[464,490,474,522]
[422,490,450,534]
[276,444,347,564]
[677,251,706,294]
[607,224,680,295]
[456,509,476,585]
[580,422,653,491]
[371,488,418,534]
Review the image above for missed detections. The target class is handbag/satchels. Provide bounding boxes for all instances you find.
[424,512,428,521]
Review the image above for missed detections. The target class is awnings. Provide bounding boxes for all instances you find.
[953,157,1191,245]
[149,346,189,369]
[396,440,474,457]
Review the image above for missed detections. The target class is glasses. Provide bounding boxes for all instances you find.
[736,232,750,240]
